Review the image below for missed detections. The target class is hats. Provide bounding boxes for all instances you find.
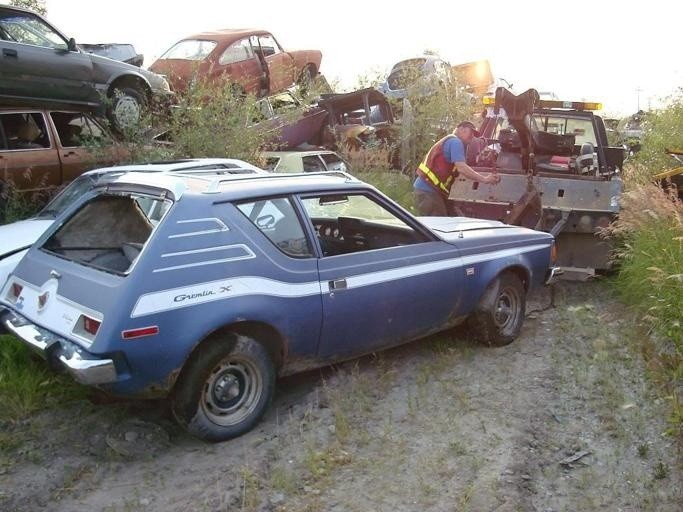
[457,120,481,138]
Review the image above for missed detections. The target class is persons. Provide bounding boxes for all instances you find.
[414,121,501,216]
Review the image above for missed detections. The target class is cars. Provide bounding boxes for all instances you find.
[2,167,562,446]
[1,155,277,338]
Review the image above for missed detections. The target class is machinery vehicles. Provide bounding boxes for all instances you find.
[439,87,634,279]
